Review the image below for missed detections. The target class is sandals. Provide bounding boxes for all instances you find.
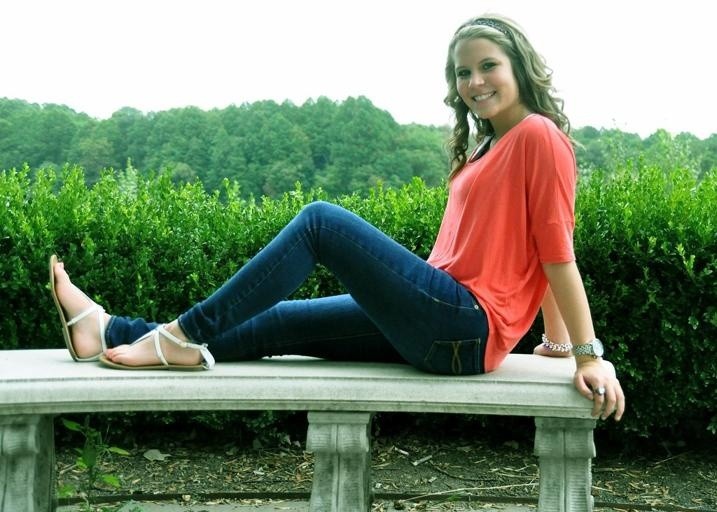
[49,255,107,363]
[98,324,215,370]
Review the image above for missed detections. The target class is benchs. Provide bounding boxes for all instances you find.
[0,347,620,512]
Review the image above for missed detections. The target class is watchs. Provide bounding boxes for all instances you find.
[570,337,605,360]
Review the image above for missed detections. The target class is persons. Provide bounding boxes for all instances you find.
[45,12,627,424]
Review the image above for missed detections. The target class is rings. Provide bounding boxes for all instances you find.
[592,385,605,395]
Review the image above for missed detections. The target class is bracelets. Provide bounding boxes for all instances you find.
[540,333,571,354]
